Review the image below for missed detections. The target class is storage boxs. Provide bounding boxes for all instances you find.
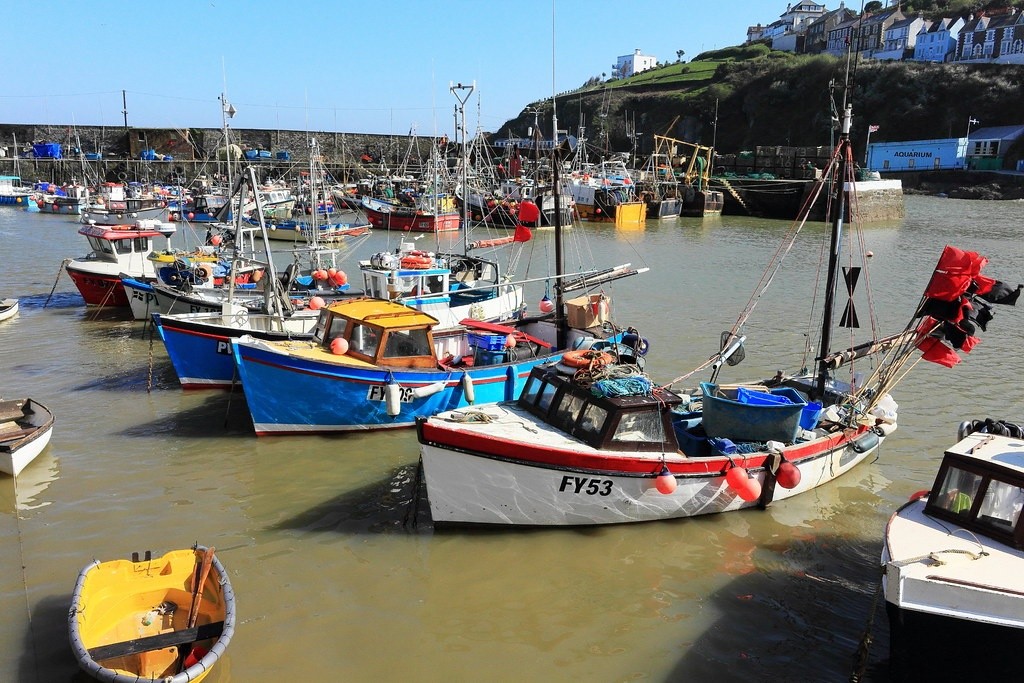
[699,381,808,442]
[566,292,612,330]
[717,146,844,182]
[467,332,505,350]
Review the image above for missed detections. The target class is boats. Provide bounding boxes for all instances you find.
[601,110,684,220]
[566,83,646,224]
[882,414,1024,683]
[67,541,235,683]
[0,298,19,321]
[0,396,54,476]
[413,21,898,531]
[64,88,527,390]
[230,60,649,438]
[642,96,724,218]
[0,90,574,243]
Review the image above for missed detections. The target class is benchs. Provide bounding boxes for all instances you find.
[0,424,43,442]
[89,616,224,662]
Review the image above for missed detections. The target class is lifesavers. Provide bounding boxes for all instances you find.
[561,349,610,368]
[174,165,185,175]
[111,225,135,230]
[400,249,432,269]
[117,171,128,181]
[644,193,653,204]
